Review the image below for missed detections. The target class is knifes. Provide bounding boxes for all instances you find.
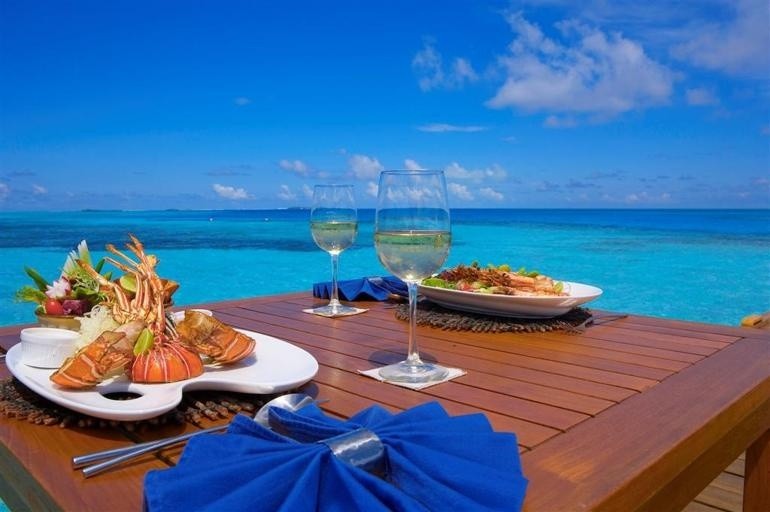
[75,396,330,468]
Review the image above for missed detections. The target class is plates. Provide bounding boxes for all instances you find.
[412,273,603,319]
[5,306,320,420]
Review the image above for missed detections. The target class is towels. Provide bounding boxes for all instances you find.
[140,401,529,512]
[312,275,410,301]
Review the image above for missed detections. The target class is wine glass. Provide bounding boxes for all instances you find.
[374,168,454,384]
[308,180,359,318]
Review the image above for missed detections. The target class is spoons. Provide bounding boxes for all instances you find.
[79,396,314,477]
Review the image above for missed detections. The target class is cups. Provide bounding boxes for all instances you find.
[21,326,78,367]
